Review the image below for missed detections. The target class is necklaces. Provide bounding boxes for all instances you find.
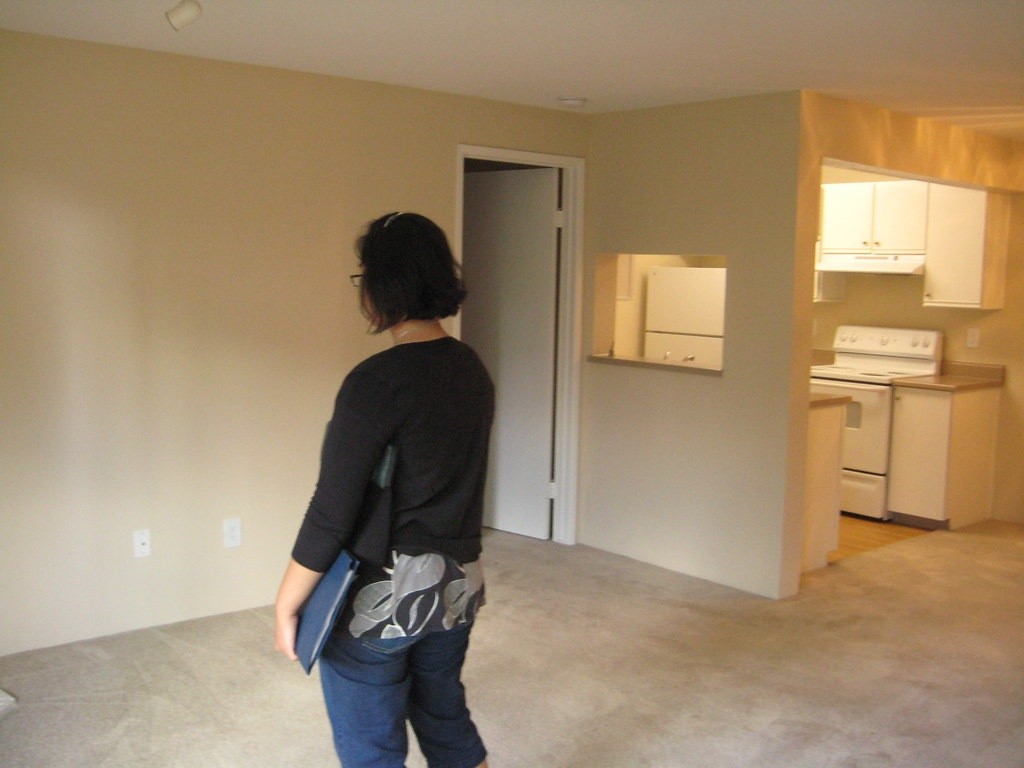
[393,320,440,342]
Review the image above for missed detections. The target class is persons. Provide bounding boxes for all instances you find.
[275,211,495,767]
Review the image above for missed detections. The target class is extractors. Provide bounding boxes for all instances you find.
[814,253,923,275]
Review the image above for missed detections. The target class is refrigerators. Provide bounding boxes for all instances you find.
[643,267,724,368]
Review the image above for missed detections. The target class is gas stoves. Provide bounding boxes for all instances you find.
[810,326,944,384]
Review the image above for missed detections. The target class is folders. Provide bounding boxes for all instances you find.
[295,550,361,676]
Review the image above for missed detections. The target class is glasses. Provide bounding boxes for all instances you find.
[350,274,367,286]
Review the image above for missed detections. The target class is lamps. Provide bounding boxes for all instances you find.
[165,1,203,31]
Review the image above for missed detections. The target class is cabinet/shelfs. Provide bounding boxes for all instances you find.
[923,182,1013,311]
[887,385,1000,529]
[819,180,927,255]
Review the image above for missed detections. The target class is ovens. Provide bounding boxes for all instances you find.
[809,379,892,520]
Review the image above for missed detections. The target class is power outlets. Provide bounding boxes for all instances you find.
[222,517,243,547]
